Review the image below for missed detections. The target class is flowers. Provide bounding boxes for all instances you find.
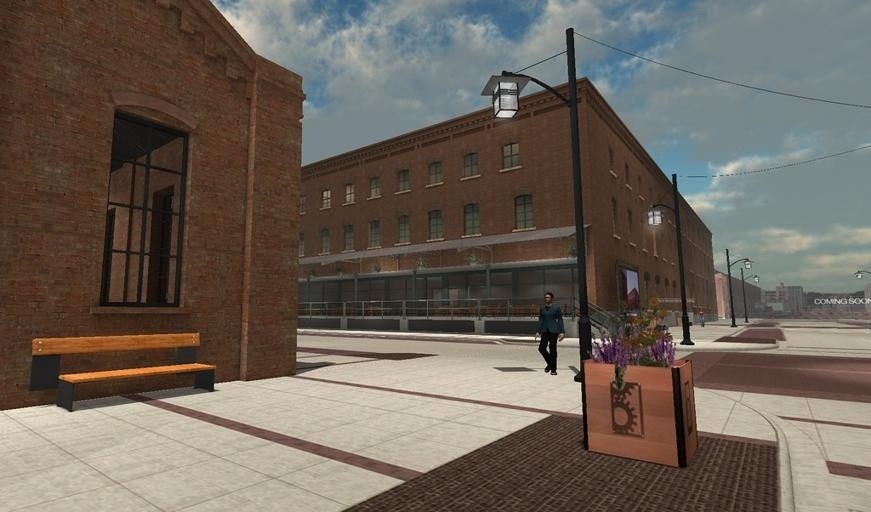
[586,294,677,390]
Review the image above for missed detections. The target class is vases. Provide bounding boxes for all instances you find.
[581,359,699,468]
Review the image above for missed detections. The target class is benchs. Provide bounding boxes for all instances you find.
[29,332,217,412]
